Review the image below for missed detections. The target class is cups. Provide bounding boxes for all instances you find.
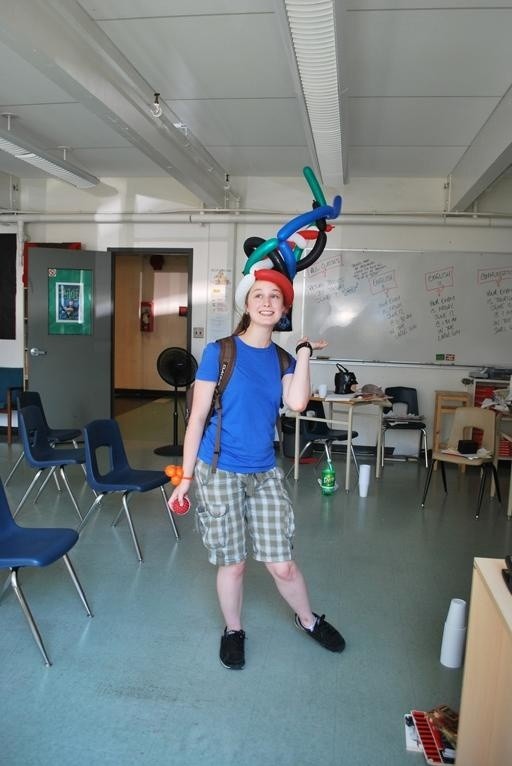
[355,384,362,394]
[358,464,371,497]
[318,383,328,398]
[440,597,467,668]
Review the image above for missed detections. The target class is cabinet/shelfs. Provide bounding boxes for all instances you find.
[468,377,512,461]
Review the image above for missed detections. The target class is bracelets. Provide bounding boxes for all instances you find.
[294,341,314,358]
[165,465,194,486]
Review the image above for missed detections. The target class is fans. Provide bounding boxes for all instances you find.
[154,347,198,457]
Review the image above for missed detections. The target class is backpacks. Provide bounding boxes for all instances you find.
[185,336,289,473]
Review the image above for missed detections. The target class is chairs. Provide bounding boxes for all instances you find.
[74,419,179,563]
[285,402,359,493]
[419,407,501,520]
[5,392,87,496]
[0,482,93,669]
[13,405,101,523]
[382,386,429,470]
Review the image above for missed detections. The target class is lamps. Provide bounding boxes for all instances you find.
[276,0,347,185]
[0,113,99,190]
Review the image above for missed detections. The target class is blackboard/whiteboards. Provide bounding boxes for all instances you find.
[301,247,512,370]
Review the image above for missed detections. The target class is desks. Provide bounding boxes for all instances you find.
[435,390,469,475]
[313,390,393,495]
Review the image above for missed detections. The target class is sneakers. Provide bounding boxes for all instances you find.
[220,626,246,669]
[294,613,346,652]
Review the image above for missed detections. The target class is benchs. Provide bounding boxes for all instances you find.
[0,368,23,446]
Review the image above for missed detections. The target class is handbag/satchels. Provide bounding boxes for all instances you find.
[334,363,358,394]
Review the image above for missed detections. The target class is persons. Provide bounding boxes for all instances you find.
[166,279,347,671]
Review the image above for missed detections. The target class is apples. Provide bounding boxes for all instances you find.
[173,499,188,514]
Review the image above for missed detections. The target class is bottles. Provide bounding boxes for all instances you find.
[173,497,192,516]
[321,458,336,496]
